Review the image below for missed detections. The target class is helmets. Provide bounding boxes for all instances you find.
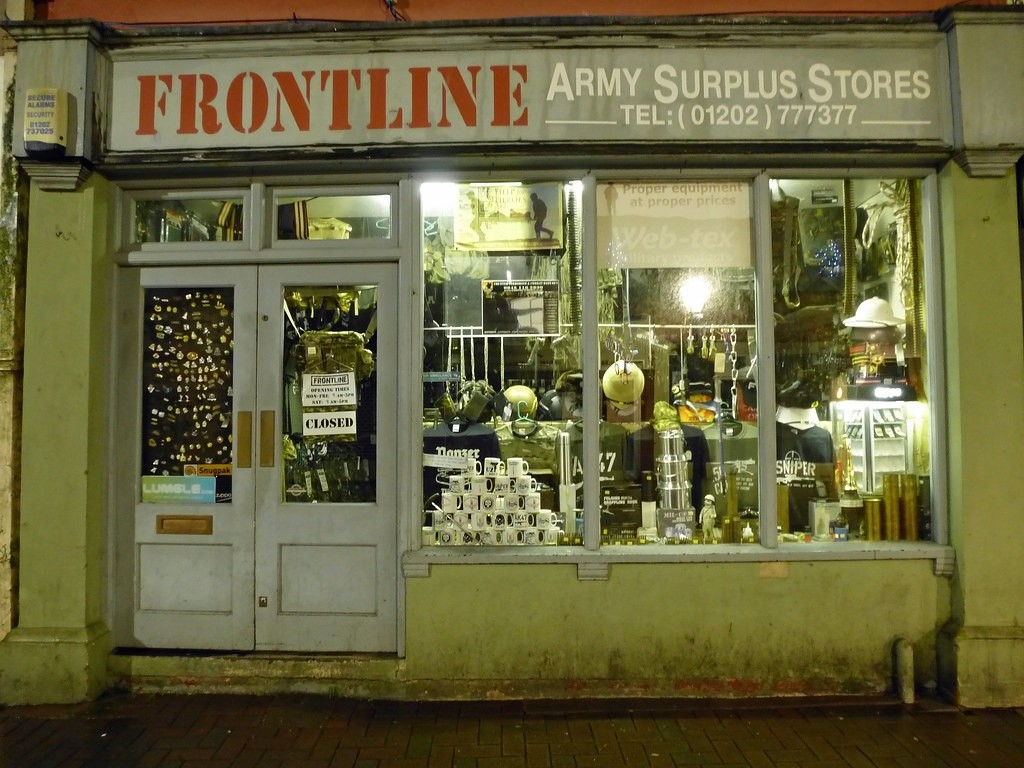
[849,328,902,344]
[503,385,538,419]
[842,296,906,328]
[603,361,645,410]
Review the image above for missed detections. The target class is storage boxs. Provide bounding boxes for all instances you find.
[481,280,563,338]
[777,479,843,541]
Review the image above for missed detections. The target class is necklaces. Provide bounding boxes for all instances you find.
[784,421,815,425]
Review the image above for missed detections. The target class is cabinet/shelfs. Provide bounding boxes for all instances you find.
[830,400,917,499]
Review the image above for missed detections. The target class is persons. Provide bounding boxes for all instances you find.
[699,494,717,539]
[775,380,837,532]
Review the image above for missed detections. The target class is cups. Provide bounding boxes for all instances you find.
[421,457,564,545]
[665,523,692,539]
